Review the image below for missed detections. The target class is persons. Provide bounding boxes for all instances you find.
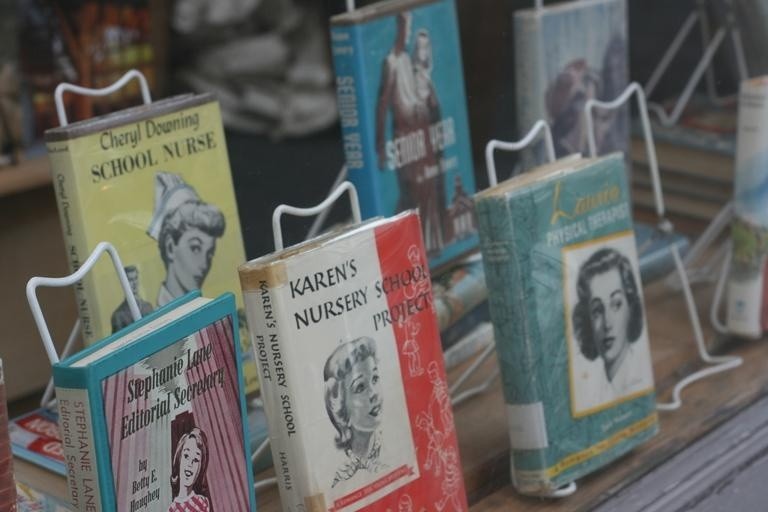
[374,9,475,262]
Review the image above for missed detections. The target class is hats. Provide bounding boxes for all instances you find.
[146,173,201,240]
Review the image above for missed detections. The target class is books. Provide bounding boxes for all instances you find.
[52,289,258,512]
[239,209,469,512]
[432,262,492,374]
[328,0,482,275]
[511,0,633,176]
[473,151,662,493]
[637,219,689,280]
[45,92,260,395]
[724,72,768,342]
[7,399,74,512]
